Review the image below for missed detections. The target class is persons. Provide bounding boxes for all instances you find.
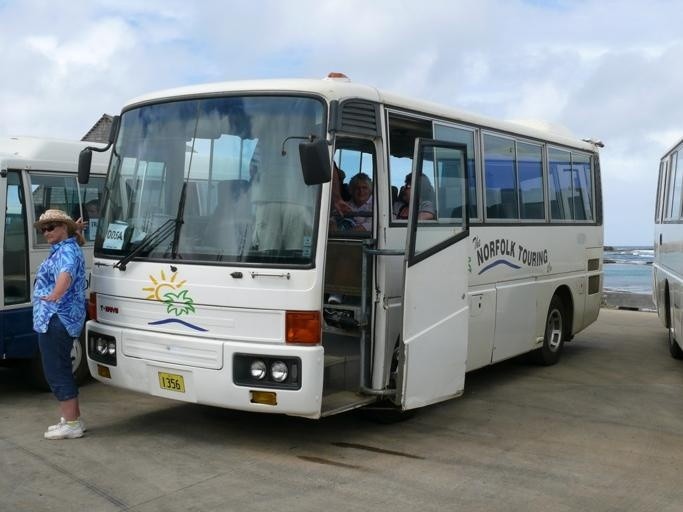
[84,199,99,220]
[249,107,353,263]
[392,172,435,221]
[31,208,91,440]
[326,172,373,305]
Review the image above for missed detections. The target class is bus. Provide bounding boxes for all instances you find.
[0,134,253,391]
[650,134,682,361]
[73,71,606,427]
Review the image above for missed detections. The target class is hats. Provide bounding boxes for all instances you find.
[32,210,78,233]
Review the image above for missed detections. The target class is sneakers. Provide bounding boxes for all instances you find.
[44,415,87,440]
[328,293,343,302]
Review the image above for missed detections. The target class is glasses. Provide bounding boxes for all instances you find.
[41,224,61,233]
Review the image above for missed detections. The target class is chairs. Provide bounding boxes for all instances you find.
[441,186,543,217]
[216,180,251,216]
